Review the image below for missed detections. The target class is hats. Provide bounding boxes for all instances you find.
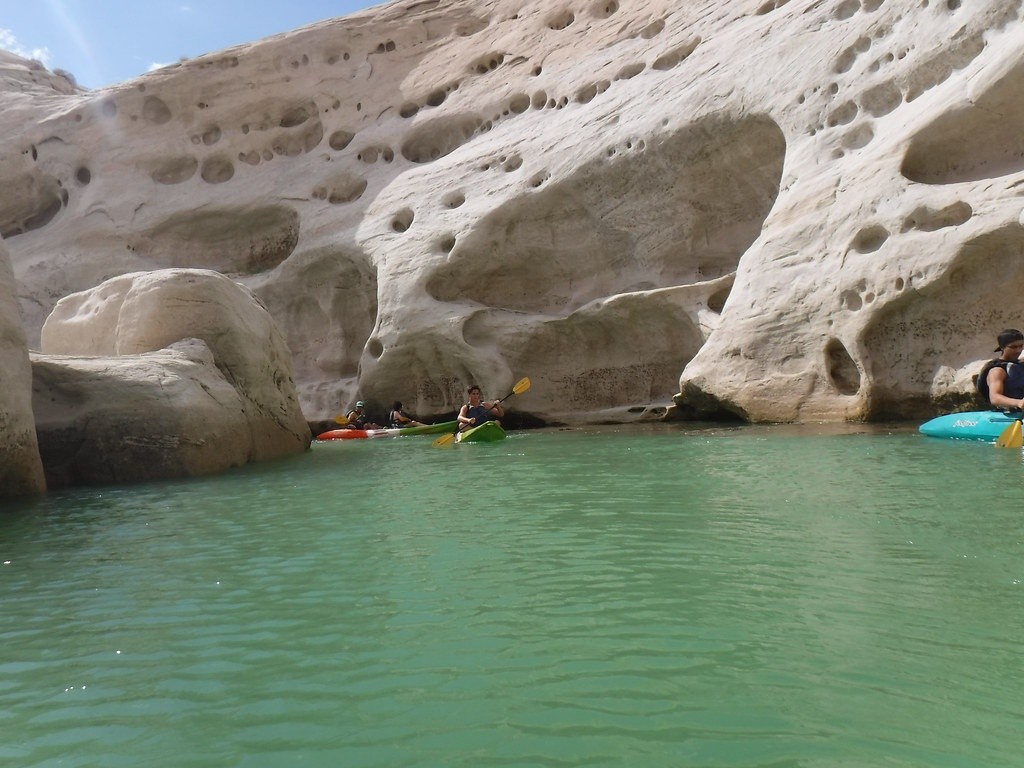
[994,329,1023,352]
[356,401,364,407]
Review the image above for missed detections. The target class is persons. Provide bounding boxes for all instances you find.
[978,328,1024,412]
[456,384,504,431]
[345,400,382,429]
[388,400,434,428]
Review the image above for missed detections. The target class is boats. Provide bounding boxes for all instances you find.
[432,421,506,448]
[918,411,1024,442]
[316,420,458,440]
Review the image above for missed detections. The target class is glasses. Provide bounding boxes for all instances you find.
[1005,344,1024,351]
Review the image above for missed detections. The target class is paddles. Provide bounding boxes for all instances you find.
[994,410,1024,448]
[434,377,531,449]
[336,415,357,424]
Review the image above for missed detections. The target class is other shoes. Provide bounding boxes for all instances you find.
[383,426,389,429]
